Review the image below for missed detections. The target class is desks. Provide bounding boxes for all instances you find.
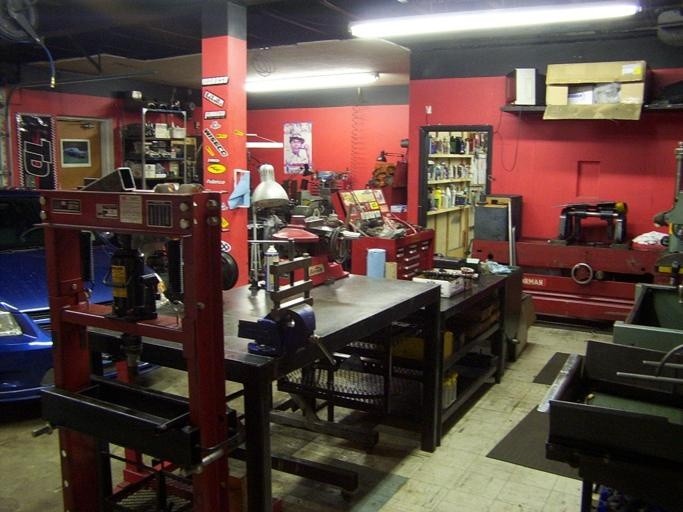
[305,262,521,442]
[86,269,440,512]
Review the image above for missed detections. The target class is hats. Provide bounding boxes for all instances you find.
[290,133,304,144]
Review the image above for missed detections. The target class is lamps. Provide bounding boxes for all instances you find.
[249,148,289,210]
[377,151,405,163]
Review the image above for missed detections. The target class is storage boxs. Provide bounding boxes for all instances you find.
[543,59,649,123]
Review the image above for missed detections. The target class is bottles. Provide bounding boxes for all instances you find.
[428,135,469,211]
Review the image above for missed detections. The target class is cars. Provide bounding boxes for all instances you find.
[0,188,166,424]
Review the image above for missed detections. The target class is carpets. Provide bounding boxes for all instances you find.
[486,351,587,484]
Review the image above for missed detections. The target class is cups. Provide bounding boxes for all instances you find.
[461,267,474,289]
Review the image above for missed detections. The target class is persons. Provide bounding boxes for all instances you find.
[285,129,310,173]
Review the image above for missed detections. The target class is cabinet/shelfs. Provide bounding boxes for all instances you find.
[120,107,187,190]
